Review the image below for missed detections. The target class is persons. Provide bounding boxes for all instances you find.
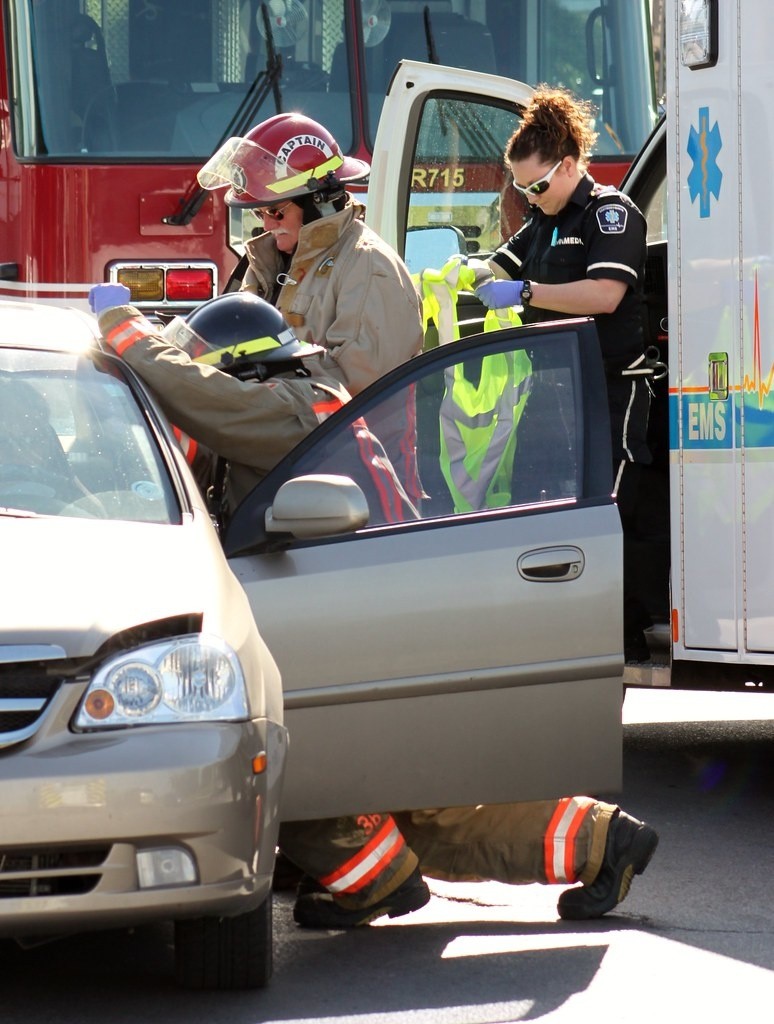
[88,282,658,927]
[448,84,671,662]
[194,112,426,528]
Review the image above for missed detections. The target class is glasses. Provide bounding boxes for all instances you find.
[512,161,563,196]
[249,202,293,222]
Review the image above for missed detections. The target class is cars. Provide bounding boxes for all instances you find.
[0,347,627,999]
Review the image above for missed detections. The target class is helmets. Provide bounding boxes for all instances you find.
[196,112,371,208]
[159,291,324,383]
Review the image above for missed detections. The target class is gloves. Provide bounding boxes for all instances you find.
[473,279,525,311]
[87,282,131,316]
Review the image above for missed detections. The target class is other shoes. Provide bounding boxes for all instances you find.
[293,866,430,929]
[557,811,661,922]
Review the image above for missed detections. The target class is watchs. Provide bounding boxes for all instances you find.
[519,279,533,308]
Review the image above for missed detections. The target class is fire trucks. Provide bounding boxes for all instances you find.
[2,0,655,531]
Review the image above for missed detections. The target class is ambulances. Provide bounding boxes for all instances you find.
[367,1,774,696]
